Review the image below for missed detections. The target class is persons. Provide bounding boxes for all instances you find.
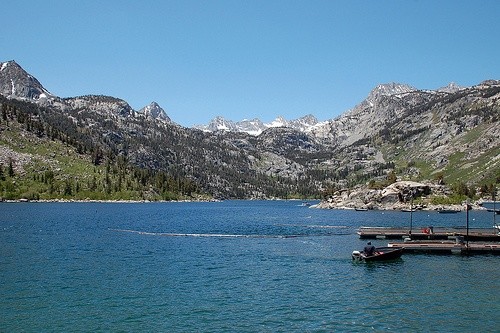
[363,241,376,257]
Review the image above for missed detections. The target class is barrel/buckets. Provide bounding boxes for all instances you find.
[456,236,464,244]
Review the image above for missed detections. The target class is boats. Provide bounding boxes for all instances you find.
[351,246,405,263]
[401,209,415,212]
[354,207,368,211]
[438,209,457,214]
